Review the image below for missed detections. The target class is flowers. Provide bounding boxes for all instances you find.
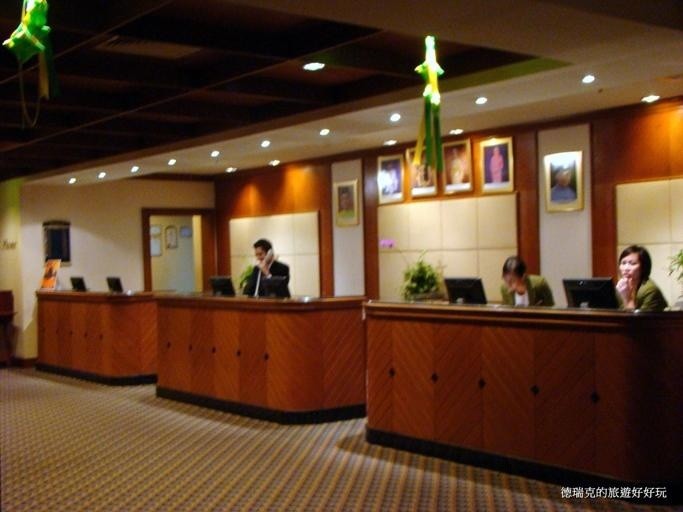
[379,238,439,301]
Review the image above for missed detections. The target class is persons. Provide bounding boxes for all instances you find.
[338,187,355,218]
[449,147,467,184]
[489,146,504,184]
[499,257,554,307]
[615,245,670,313]
[382,159,399,194]
[416,154,432,188]
[241,237,290,298]
[551,165,577,204]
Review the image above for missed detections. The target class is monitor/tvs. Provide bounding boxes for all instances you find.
[106,276,121,291]
[70,276,86,290]
[208,277,236,295]
[561,278,619,309]
[443,277,486,305]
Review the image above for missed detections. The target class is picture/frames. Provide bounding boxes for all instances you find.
[333,178,361,227]
[405,145,441,199]
[543,150,585,214]
[440,137,475,196]
[478,135,516,195]
[377,154,408,204]
[149,224,193,258]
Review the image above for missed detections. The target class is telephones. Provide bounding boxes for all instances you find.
[263,248,273,265]
